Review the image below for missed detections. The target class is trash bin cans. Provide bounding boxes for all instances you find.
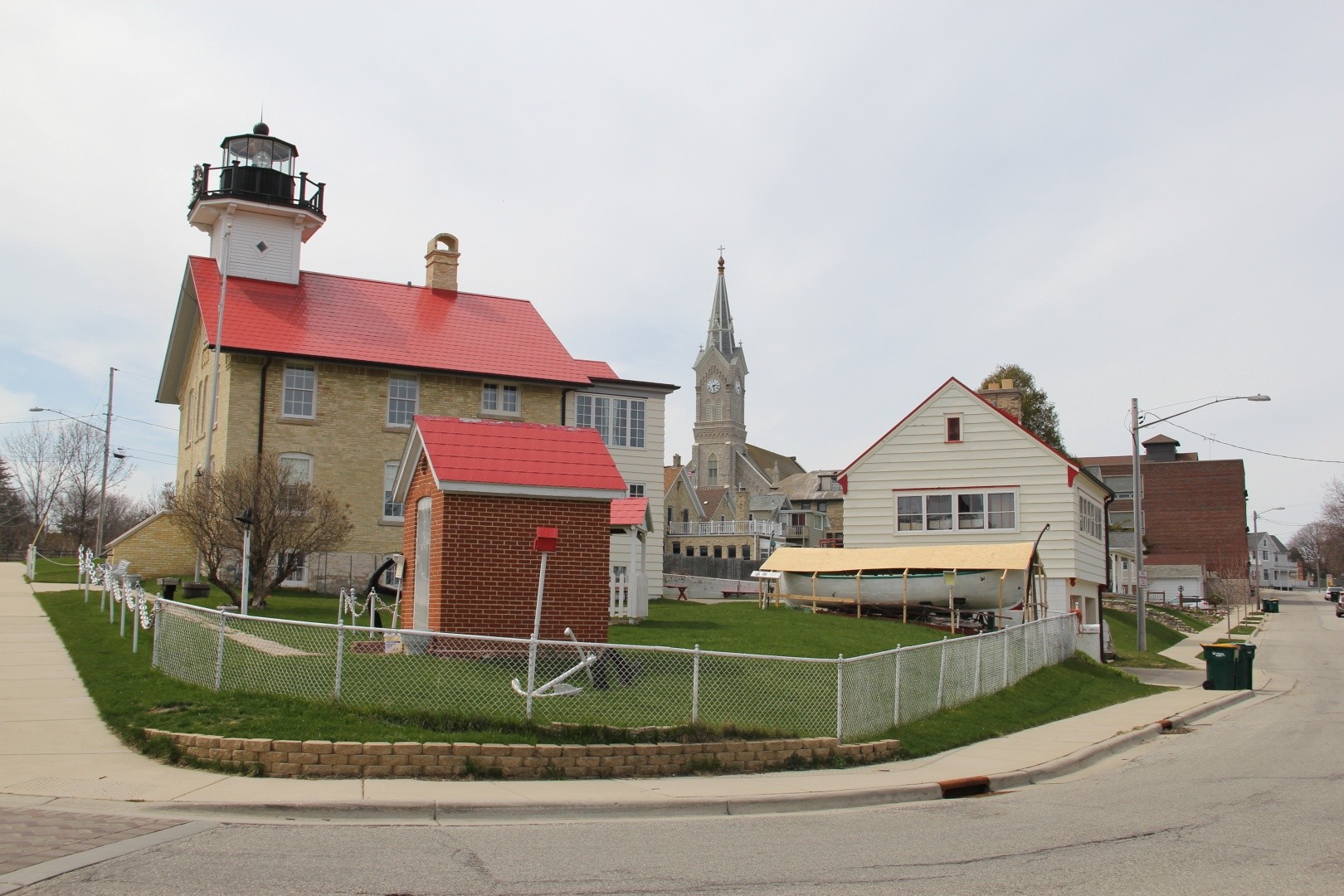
[1261,599,1279,613]
[1199,643,1257,690]
[1331,591,1340,603]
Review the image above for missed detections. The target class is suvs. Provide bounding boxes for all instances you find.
[1165,596,1210,610]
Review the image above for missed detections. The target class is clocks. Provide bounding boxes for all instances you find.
[734,381,742,395]
[706,378,721,394]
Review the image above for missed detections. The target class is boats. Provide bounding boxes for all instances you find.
[779,567,1025,618]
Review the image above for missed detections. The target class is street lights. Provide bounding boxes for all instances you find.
[30,406,110,558]
[1134,394,1271,651]
[1253,507,1286,609]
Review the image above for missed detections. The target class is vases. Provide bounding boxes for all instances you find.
[399,629,435,657]
[122,574,143,590]
[182,581,211,599]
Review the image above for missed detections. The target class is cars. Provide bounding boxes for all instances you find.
[1323,585,1343,601]
[1336,597,1344,617]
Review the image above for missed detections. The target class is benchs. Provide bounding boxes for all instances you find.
[719,591,764,600]
[112,558,133,581]
[663,584,688,601]
